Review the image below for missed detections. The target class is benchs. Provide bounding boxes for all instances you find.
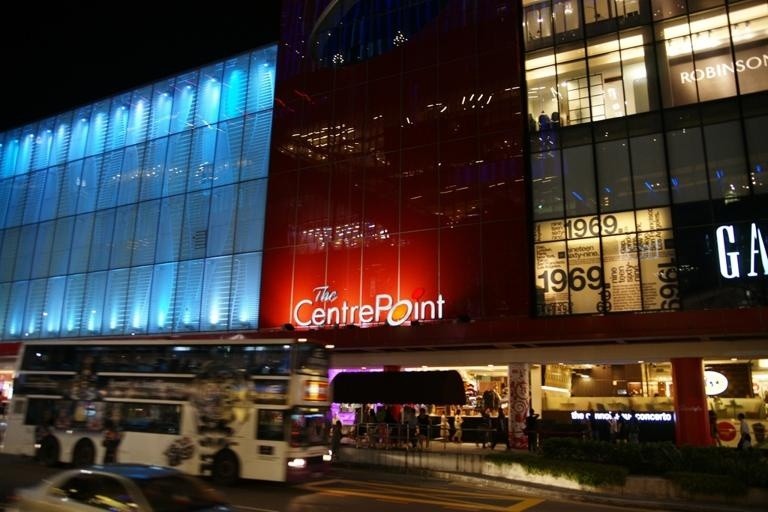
[428,415,508,444]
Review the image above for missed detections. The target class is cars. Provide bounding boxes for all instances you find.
[8,464,259,512]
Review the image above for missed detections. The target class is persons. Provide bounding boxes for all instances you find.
[475,407,490,447]
[354,405,465,448]
[582,409,640,447]
[526,409,539,452]
[710,410,721,447]
[489,408,509,449]
[539,110,550,130]
[737,413,751,448]
[328,421,348,463]
[529,113,535,130]
[103,430,122,462]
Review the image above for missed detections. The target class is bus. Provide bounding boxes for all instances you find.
[3,337,334,486]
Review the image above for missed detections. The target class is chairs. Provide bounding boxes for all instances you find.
[357,423,416,451]
